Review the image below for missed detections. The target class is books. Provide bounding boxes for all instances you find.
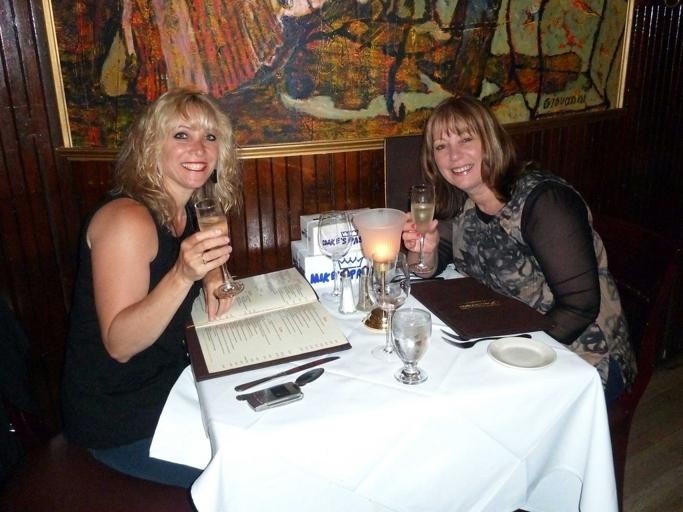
[402,276,555,340]
[183,265,352,382]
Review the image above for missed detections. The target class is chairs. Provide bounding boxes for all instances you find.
[1,294,199,512]
[591,213,678,512]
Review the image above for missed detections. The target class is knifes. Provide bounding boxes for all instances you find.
[234,355,344,393]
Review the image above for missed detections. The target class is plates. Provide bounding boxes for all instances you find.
[484,334,558,370]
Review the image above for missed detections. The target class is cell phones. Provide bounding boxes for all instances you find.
[247,382,303,412]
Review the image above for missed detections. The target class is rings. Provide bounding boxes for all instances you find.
[200,251,207,265]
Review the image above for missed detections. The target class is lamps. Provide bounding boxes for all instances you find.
[351,207,408,332]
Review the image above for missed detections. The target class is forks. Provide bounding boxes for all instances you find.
[440,326,533,349]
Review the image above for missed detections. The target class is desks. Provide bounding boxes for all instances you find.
[186,252,600,511]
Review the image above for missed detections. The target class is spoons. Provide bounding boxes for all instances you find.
[235,366,324,402]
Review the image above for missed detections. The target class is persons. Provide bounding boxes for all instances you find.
[56,84,236,489]
[402,93,638,411]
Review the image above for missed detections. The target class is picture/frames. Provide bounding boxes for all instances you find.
[38,1,636,163]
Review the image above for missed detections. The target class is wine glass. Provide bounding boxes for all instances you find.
[195,197,246,301]
[410,182,438,275]
[316,210,432,386]
